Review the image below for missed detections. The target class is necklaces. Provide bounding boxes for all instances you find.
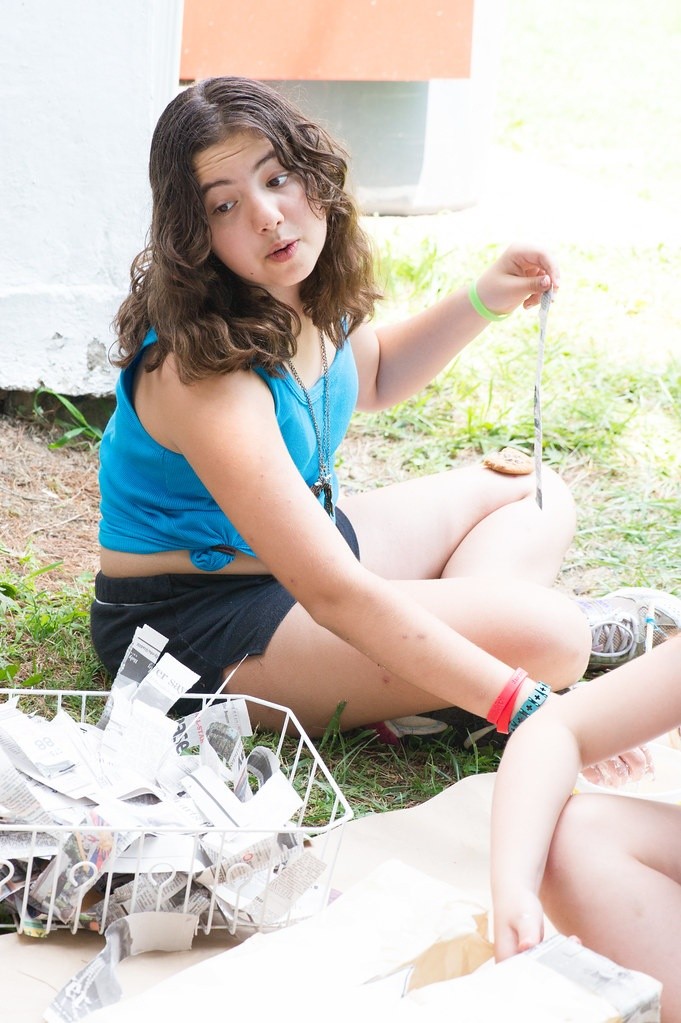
[285,320,333,487]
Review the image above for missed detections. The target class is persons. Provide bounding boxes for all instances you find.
[462,626,680,1021]
[83,72,679,784]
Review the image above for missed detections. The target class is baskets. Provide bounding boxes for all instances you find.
[0,688,354,933]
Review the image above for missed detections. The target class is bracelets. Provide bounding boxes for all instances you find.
[507,680,551,736]
[497,676,527,734]
[468,282,513,323]
[485,665,529,724]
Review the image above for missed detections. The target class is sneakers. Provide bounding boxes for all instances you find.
[361,706,514,753]
[567,585,681,671]
